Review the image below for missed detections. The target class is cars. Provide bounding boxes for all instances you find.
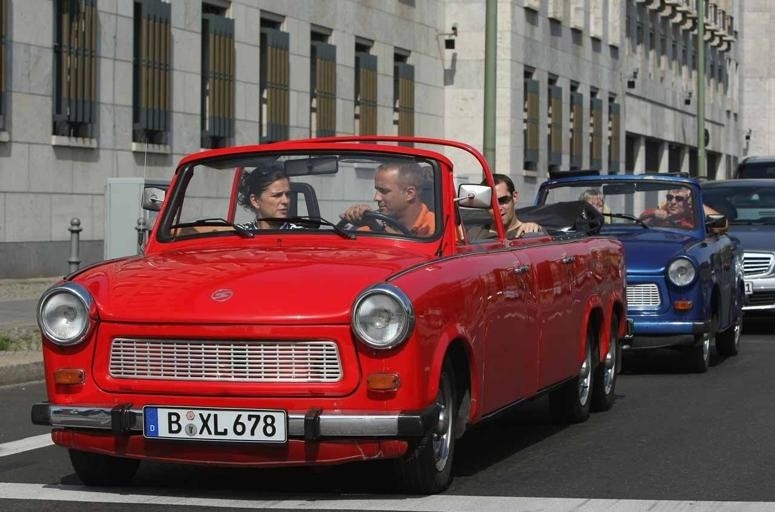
[677,176,775,332]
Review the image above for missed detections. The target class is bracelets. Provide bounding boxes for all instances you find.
[369,220,388,232]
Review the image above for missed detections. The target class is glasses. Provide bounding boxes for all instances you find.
[666,194,685,202]
[498,196,514,205]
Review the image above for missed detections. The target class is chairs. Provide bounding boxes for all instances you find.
[289,166,434,227]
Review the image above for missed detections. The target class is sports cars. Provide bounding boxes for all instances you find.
[28,134,630,502]
[531,167,745,375]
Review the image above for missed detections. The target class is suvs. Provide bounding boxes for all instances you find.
[733,154,775,182]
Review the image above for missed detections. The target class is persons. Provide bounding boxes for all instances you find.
[638,185,728,235]
[578,189,606,223]
[456,174,549,242]
[338,157,437,239]
[232,162,308,230]
